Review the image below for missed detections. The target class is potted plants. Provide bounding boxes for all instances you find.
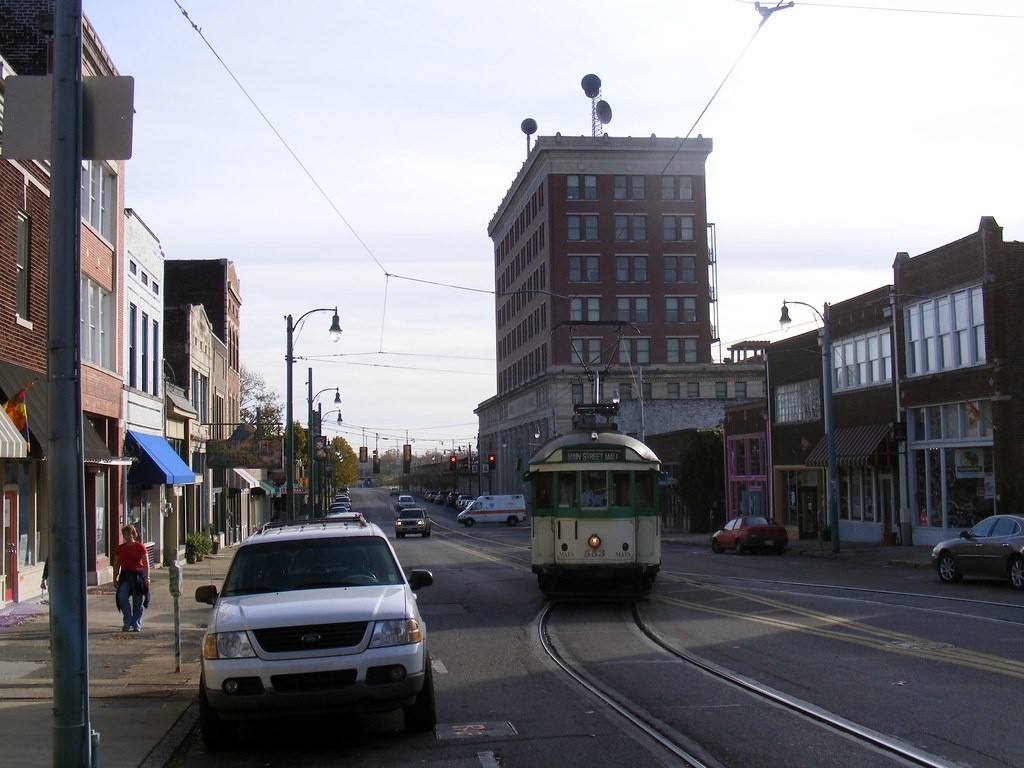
[186,532,212,563]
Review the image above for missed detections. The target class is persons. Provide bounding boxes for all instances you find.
[112,524,151,632]
[40,554,49,590]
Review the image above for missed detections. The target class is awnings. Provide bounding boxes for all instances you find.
[232,469,261,489]
[0,404,27,458]
[805,423,893,467]
[252,479,280,496]
[126,429,195,485]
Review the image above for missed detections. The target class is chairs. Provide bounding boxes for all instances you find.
[245,560,282,591]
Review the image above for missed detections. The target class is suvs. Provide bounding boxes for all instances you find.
[195,514,438,754]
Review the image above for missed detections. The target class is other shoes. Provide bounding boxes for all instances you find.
[134,627,141,631]
[122,616,133,631]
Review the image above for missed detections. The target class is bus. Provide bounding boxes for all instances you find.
[522,424,668,606]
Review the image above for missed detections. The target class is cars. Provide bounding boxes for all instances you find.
[424,490,475,511]
[712,516,789,556]
[931,514,1024,590]
[396,507,432,539]
[390,488,401,497]
[324,487,366,521]
[396,495,417,512]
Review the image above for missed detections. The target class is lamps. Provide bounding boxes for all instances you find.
[535,427,541,438]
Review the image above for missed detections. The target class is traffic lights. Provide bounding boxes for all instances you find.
[515,457,522,471]
[488,453,496,470]
[450,456,457,471]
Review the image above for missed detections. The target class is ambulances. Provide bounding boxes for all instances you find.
[456,494,527,527]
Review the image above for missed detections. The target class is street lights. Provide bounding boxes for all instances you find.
[779,302,841,554]
[534,406,557,439]
[308,368,342,519]
[317,403,343,517]
[287,306,342,525]
[611,366,645,444]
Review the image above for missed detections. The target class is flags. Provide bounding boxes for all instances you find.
[2,382,34,452]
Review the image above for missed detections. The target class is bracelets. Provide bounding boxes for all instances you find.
[143,576,150,579]
